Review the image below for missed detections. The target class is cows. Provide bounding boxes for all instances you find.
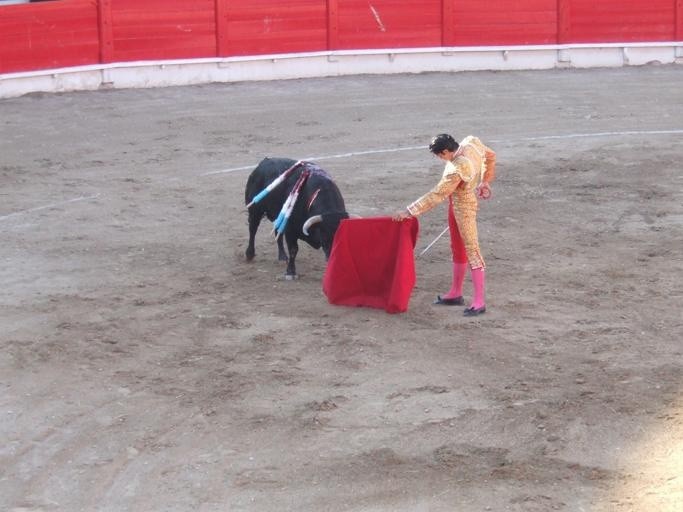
[244,156,365,283]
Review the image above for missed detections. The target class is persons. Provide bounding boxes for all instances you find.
[393,134,498,318]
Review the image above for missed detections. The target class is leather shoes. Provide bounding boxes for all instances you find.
[433,296,465,305]
[463,305,486,316]
